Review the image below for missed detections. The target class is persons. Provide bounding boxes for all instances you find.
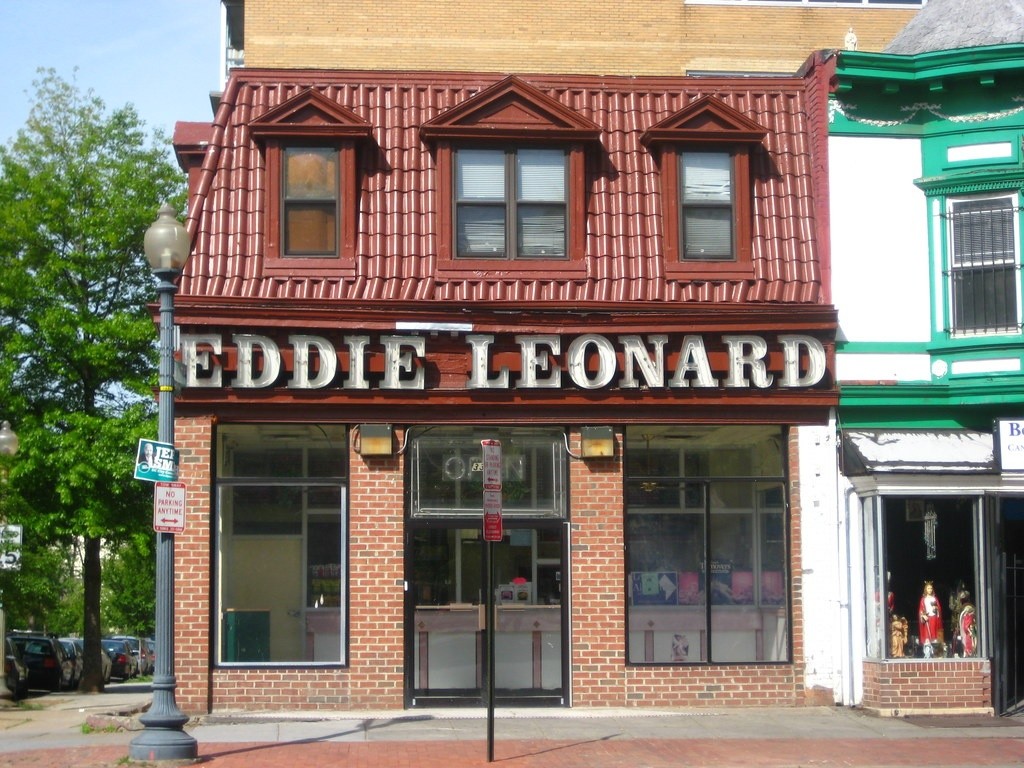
[918,580,945,658]
[138,443,157,466]
[889,613,910,658]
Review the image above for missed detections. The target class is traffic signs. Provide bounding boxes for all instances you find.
[481,439,503,543]
[153,480,187,535]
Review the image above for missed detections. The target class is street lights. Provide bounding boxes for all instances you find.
[127,201,201,758]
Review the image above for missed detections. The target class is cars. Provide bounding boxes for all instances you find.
[100,639,139,680]
[112,636,151,674]
[75,637,114,681]
[142,639,157,669]
[10,629,73,692]
[53,638,85,684]
[4,636,30,696]
